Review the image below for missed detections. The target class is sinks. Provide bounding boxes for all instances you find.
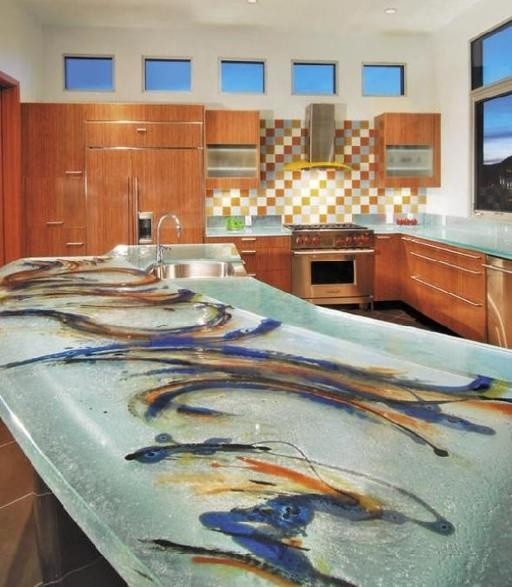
[144,260,230,280]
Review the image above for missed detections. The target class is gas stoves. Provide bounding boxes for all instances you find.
[285,219,372,247]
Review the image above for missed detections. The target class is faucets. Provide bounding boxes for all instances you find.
[155,213,182,263]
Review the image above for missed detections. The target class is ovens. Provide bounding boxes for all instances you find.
[293,248,374,310]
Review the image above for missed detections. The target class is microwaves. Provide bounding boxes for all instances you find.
[384,145,433,179]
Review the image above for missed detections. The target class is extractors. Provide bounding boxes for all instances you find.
[280,103,354,173]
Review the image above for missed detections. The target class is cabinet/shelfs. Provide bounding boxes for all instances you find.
[399,232,489,345]
[204,236,293,293]
[85,102,204,255]
[373,112,441,188]
[204,109,261,190]
[374,234,399,302]
[20,102,85,256]
